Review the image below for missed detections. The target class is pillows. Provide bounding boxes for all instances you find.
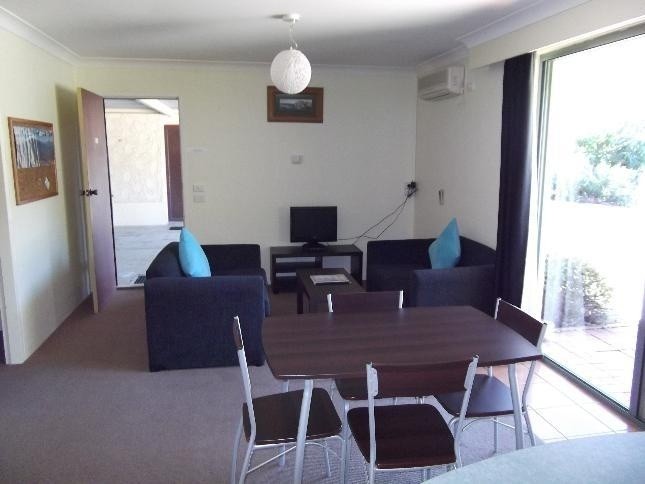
[178,227,212,278]
[427,218,462,269]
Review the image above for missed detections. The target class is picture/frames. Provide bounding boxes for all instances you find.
[7,116,59,206]
[265,84,324,124]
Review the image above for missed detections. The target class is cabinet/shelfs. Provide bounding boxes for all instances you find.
[269,243,363,295]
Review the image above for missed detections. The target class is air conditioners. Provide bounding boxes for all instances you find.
[417,65,466,102]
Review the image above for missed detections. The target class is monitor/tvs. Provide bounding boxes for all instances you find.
[289,206,338,249]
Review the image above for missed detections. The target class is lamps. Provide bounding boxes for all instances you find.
[270,13,312,95]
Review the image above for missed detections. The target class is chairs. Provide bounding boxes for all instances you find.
[423,297,547,473]
[231,316,347,484]
[326,289,423,440]
[347,351,480,484]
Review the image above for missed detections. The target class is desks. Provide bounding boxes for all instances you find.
[260,304,543,484]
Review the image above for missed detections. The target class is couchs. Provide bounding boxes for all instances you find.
[142,241,271,373]
[365,234,497,318]
